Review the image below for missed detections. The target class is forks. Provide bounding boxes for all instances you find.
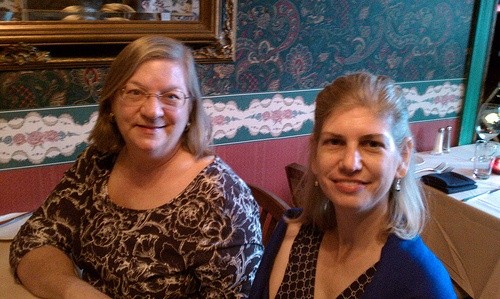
[416,167,454,180]
[413,162,446,173]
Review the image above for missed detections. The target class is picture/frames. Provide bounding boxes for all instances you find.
[0,0,237,72]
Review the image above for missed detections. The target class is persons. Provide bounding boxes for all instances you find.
[9,35,265,299]
[247,73,459,299]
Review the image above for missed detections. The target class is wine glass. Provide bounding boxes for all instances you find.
[475,102,500,160]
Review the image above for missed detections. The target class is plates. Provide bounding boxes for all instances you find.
[412,154,424,165]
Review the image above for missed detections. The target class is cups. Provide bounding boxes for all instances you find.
[472,140,498,181]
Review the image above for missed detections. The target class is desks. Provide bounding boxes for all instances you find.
[405,141,500,299]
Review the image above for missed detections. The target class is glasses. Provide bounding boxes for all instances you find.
[118,83,192,110]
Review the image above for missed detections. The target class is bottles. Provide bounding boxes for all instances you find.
[430,125,452,155]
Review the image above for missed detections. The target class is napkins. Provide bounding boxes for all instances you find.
[422,172,478,194]
